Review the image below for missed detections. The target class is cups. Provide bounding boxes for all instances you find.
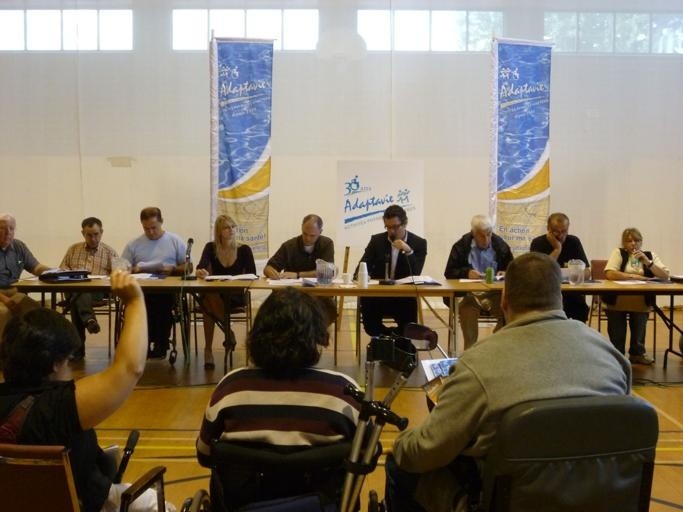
[111,258,132,274]
[342,273,352,285]
[663,267,671,280]
[568,259,591,286]
[358,262,368,289]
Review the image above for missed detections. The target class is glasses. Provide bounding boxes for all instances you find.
[384,223,403,231]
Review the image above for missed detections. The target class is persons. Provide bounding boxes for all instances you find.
[382,251,633,504]
[353,205,427,339]
[1,215,52,328]
[529,213,590,326]
[264,215,337,330]
[600,229,670,366]
[57,216,120,357]
[1,269,177,512]
[119,207,187,358]
[196,216,256,372]
[443,215,514,350]
[193,286,368,501]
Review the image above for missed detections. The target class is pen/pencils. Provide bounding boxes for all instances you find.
[280,268,284,274]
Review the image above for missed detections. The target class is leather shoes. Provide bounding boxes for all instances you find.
[205,363,215,369]
[87,319,100,333]
[629,353,655,365]
[223,341,236,351]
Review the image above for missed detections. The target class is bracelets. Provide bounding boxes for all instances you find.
[404,250,412,253]
[647,261,653,268]
[297,272,299,279]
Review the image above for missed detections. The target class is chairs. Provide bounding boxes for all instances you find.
[0,420,169,511]
[179,441,366,511]
[492,396,659,512]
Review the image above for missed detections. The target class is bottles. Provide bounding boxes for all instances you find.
[631,249,639,268]
[486,262,494,284]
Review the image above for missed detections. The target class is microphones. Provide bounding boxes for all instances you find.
[389,234,409,254]
[183,237,195,263]
